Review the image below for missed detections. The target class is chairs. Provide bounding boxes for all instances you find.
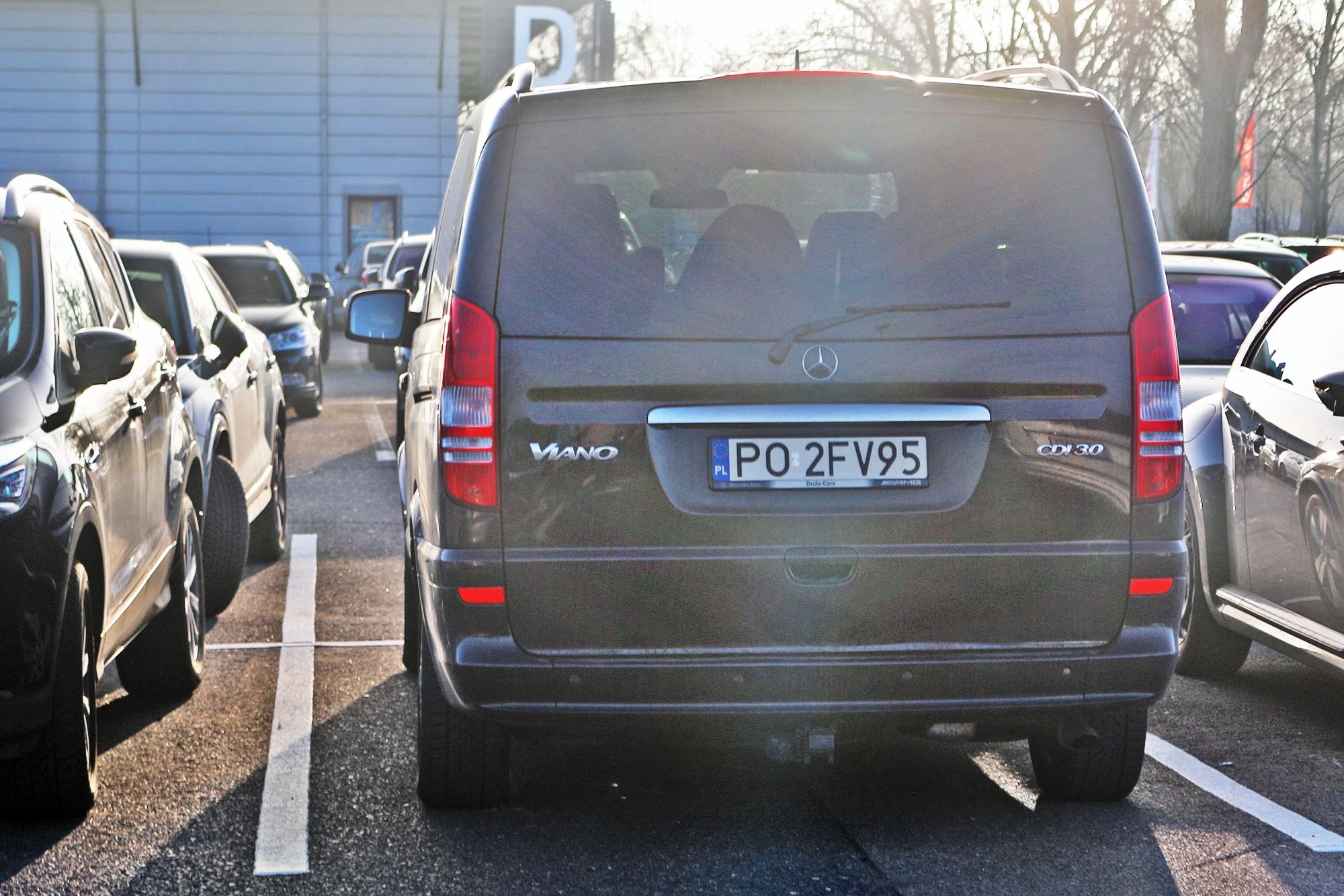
[506,184,1072,315]
[1179,313,1230,352]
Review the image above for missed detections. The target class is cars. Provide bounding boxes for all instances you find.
[109,236,294,616]
[1159,227,1344,691]
[0,167,209,836]
[189,230,434,422]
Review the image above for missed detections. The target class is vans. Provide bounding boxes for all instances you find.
[339,61,1197,805]
